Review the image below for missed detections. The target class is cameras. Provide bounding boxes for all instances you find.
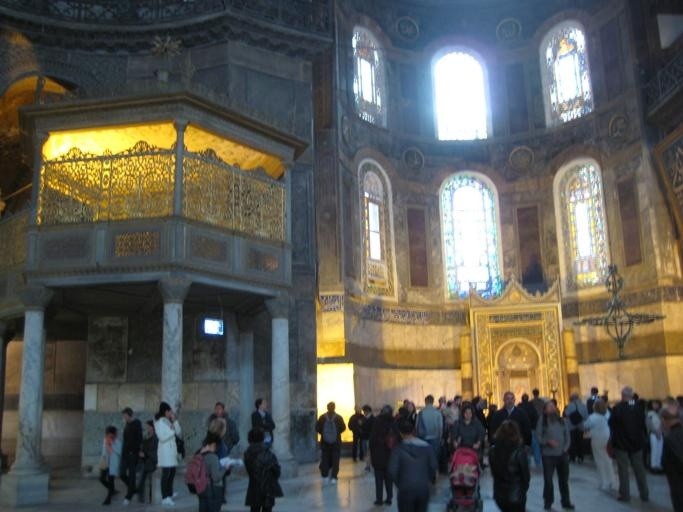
[592,395,598,400]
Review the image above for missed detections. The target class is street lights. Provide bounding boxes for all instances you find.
[548,385,557,401]
[483,388,493,408]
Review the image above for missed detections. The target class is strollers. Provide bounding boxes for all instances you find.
[446,443,484,512]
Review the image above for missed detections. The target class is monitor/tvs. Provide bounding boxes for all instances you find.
[202,316,225,336]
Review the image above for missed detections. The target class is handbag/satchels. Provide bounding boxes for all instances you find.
[568,410,583,425]
[175,434,185,460]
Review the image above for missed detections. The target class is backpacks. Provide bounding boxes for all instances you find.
[183,449,212,495]
[322,413,338,444]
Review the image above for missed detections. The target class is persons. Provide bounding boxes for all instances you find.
[96,397,283,511]
[316,387,683,511]
